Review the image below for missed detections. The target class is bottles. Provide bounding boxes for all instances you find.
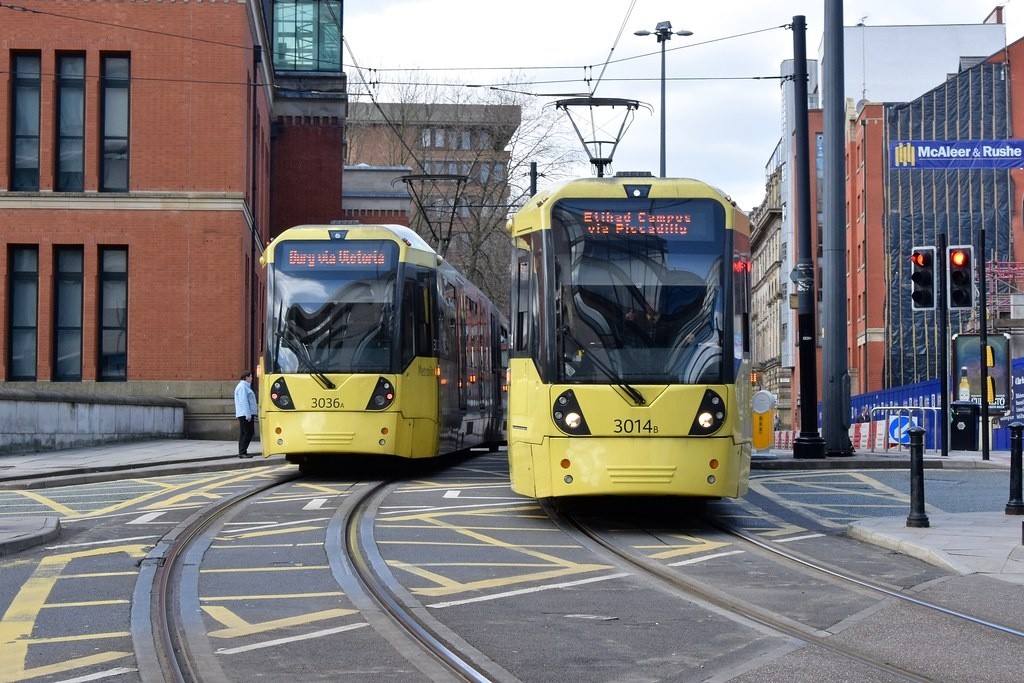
[958,365,970,401]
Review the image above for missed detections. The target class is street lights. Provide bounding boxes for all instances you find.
[634,21,693,178]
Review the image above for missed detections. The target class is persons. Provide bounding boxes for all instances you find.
[856,407,870,423]
[234,371,257,458]
[625,294,670,348]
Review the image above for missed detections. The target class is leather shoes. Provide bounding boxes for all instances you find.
[238,454,254,459]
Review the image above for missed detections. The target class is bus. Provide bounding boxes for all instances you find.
[504,97,776,500]
[256,173,511,460]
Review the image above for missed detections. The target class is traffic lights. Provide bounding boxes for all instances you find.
[945,245,975,310]
[911,247,937,310]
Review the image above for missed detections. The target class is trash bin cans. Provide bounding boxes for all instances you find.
[950,401,980,451]
